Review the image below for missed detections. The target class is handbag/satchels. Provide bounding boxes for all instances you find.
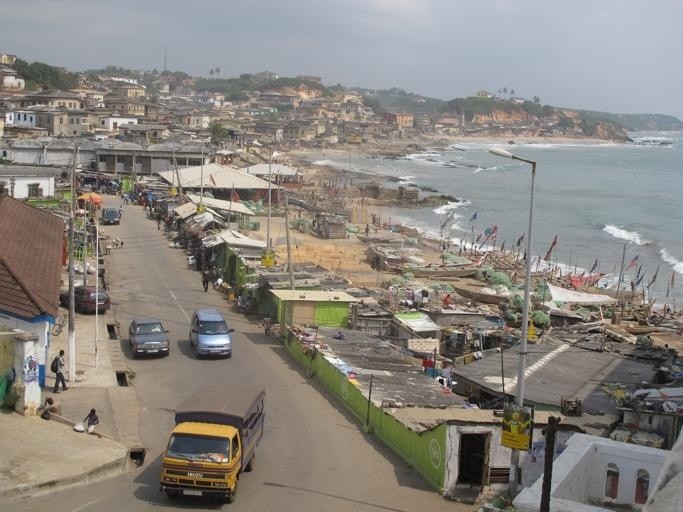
[73,422,85,432]
[42,411,50,420]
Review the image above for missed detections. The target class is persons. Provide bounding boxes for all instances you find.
[246,289,254,308]
[84,408,99,428]
[44,398,63,416]
[261,313,270,336]
[186,254,195,268]
[237,287,244,306]
[156,211,163,229]
[202,267,210,292]
[118,205,124,218]
[212,263,218,278]
[443,293,450,305]
[53,349,69,393]
[121,192,153,210]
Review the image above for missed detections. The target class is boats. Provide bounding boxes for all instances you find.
[405,260,477,278]
[451,231,681,337]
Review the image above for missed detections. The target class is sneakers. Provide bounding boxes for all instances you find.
[53,387,69,393]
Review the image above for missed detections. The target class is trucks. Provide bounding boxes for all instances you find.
[159,386,265,504]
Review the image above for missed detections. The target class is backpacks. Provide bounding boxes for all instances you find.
[51,356,61,373]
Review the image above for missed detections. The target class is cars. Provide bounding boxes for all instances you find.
[128,318,169,358]
[102,207,119,224]
[59,285,110,313]
[189,309,233,359]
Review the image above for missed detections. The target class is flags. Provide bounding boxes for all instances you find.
[441,210,675,298]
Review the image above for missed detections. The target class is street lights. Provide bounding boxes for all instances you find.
[487,144,537,498]
[257,138,272,254]
